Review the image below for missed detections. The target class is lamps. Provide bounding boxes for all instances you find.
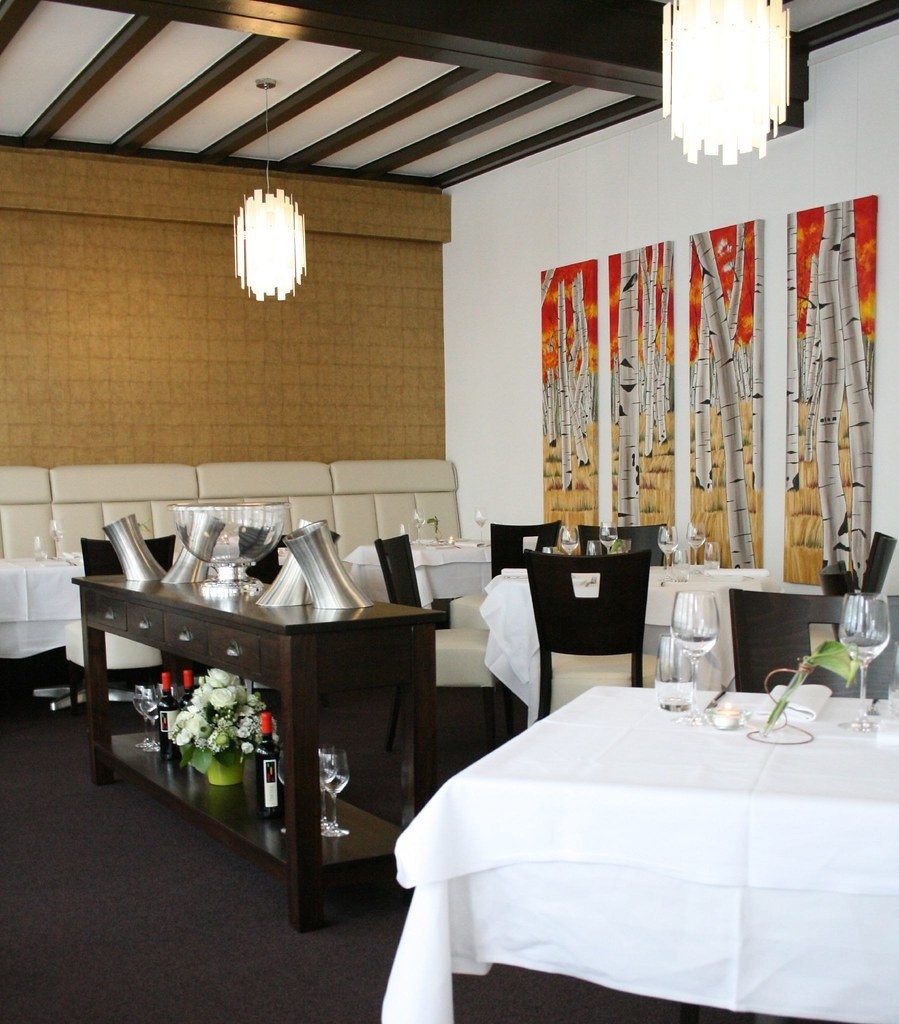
[232,78,307,302]
[662,0,790,167]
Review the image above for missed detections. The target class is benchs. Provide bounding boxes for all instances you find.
[0,457,461,560]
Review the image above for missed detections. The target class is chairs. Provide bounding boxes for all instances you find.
[728,587,899,700]
[861,532,897,597]
[818,560,860,643]
[523,549,654,721]
[491,519,563,580]
[373,537,506,754]
[35,534,175,712]
[239,527,342,586]
[578,525,668,567]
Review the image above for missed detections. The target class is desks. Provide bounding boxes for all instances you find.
[72,575,446,935]
[479,566,772,731]
[341,539,491,611]
[0,557,85,659]
[380,684,899,1024]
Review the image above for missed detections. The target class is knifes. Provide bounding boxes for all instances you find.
[704,691,726,711]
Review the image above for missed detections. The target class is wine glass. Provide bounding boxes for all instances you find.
[49,519,67,561]
[838,592,891,732]
[133,684,161,752]
[685,522,706,576]
[277,744,351,837]
[657,526,679,581]
[558,525,580,555]
[671,590,720,726]
[473,507,490,544]
[410,508,426,543]
[599,521,618,555]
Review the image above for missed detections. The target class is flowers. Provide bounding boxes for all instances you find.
[168,668,281,776]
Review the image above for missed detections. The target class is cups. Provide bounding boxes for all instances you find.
[671,544,690,582]
[542,546,559,554]
[400,523,411,543]
[586,540,603,555]
[654,633,698,713]
[888,641,899,720]
[152,684,179,705]
[33,536,49,562]
[703,542,721,578]
[618,539,632,554]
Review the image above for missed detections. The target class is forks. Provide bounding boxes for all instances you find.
[867,698,880,716]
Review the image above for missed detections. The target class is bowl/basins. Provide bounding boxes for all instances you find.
[704,708,752,730]
[167,500,293,590]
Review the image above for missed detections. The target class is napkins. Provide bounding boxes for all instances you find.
[758,685,834,722]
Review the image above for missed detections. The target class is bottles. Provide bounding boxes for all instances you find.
[254,712,285,820]
[157,672,181,760]
[179,670,199,710]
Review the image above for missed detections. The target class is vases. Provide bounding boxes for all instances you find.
[204,753,242,786]
